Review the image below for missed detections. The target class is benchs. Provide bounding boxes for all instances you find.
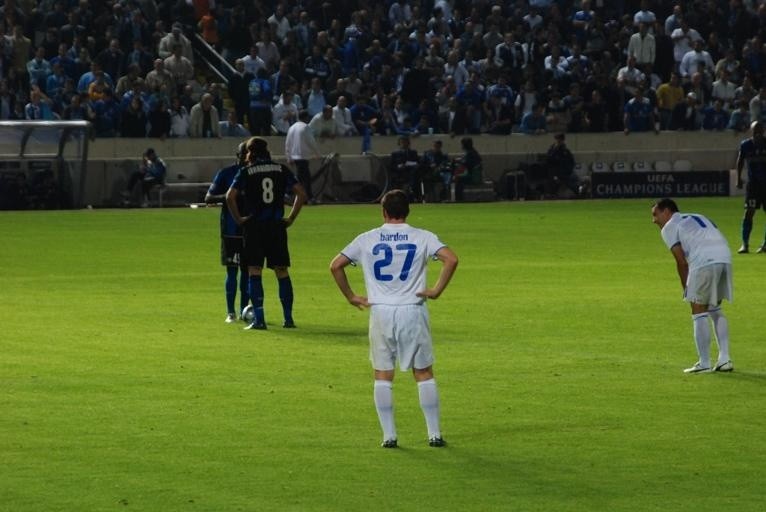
[137,178,211,210]
[417,174,496,204]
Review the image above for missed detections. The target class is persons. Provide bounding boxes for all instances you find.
[1,2,766,208]
[736,121,766,252]
[225,138,306,329]
[204,141,250,323]
[652,198,733,373]
[330,189,458,447]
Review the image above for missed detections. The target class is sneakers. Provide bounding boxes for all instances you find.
[225,312,296,329]
[683,360,734,373]
